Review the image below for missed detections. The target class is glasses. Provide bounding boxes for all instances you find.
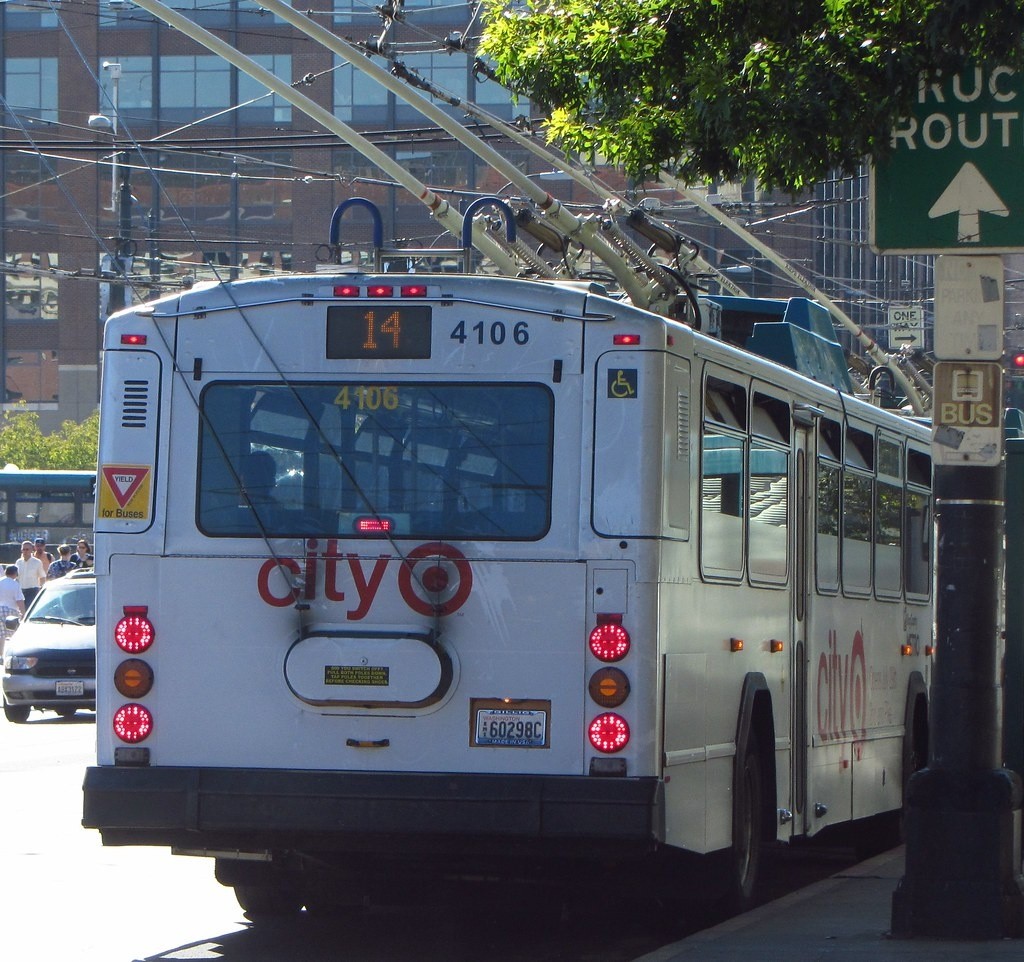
[78,546,86,549]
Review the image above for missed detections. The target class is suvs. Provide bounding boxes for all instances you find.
[2,566,96,724]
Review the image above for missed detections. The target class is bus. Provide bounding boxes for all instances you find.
[82,1,939,917]
[0,463,95,566]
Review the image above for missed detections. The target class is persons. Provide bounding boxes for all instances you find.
[239,450,287,512]
[0,538,96,671]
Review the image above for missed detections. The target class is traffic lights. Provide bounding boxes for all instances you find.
[1010,348,1024,375]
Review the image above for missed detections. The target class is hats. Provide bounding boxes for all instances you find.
[35,538,45,545]
